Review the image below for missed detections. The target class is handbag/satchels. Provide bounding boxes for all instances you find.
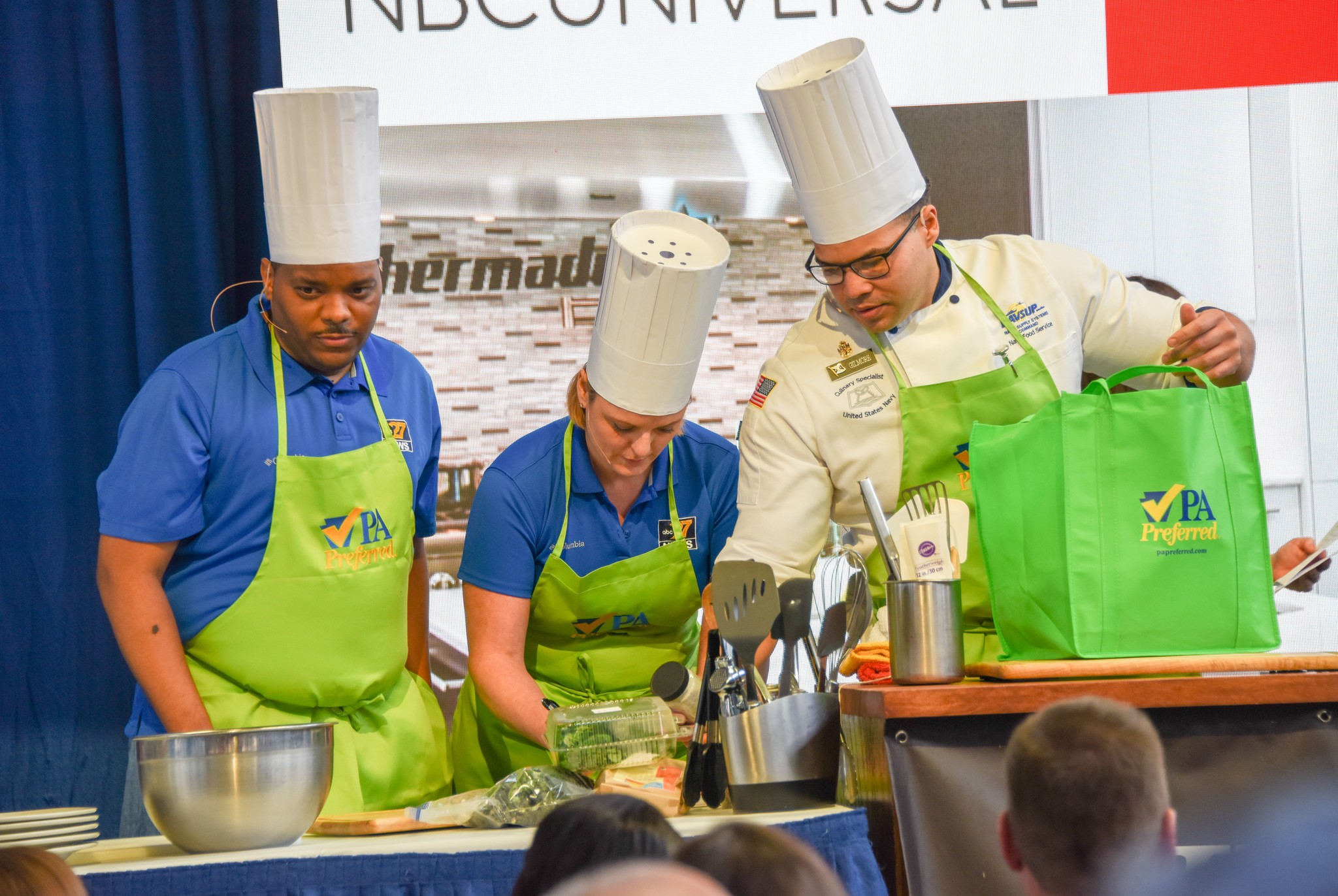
[968,364,1280,663]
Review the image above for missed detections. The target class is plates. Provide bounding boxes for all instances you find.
[0,807,102,864]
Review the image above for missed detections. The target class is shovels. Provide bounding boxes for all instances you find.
[711,559,781,700]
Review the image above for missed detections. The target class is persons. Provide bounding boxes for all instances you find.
[1082,270,1332,594]
[453,358,742,796]
[704,172,1255,687]
[997,700,1180,896]
[0,792,853,896]
[93,218,456,837]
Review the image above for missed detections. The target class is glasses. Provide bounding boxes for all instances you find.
[804,212,921,285]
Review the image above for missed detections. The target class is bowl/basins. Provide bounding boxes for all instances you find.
[718,688,841,814]
[880,580,965,685]
[133,720,341,853]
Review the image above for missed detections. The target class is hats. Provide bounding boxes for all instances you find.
[584,210,731,416]
[251,83,380,264]
[754,37,930,244]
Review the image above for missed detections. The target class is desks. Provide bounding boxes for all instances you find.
[841,651,1338,895]
[89,802,888,896]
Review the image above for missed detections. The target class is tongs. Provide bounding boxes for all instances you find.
[682,629,728,809]
[858,476,903,581]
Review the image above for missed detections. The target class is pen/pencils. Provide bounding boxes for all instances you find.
[541,698,561,712]
